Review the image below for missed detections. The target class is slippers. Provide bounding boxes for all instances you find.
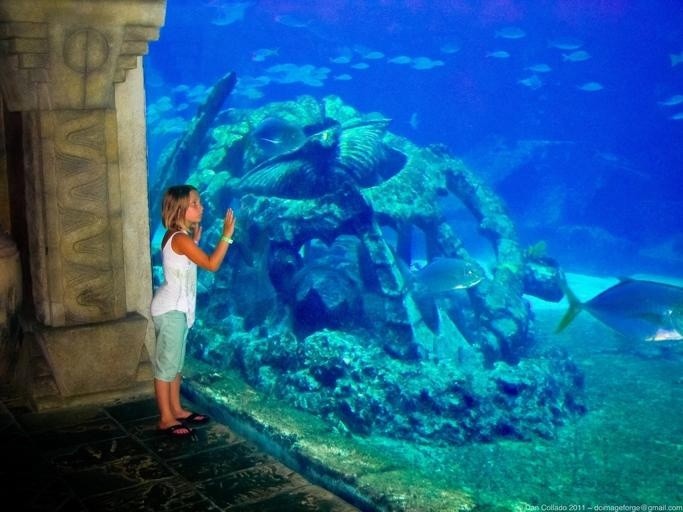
[156,420,193,436]
[173,409,210,426]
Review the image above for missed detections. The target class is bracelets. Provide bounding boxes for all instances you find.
[221,235,233,244]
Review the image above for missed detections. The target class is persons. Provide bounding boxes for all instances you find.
[150,185,236,438]
